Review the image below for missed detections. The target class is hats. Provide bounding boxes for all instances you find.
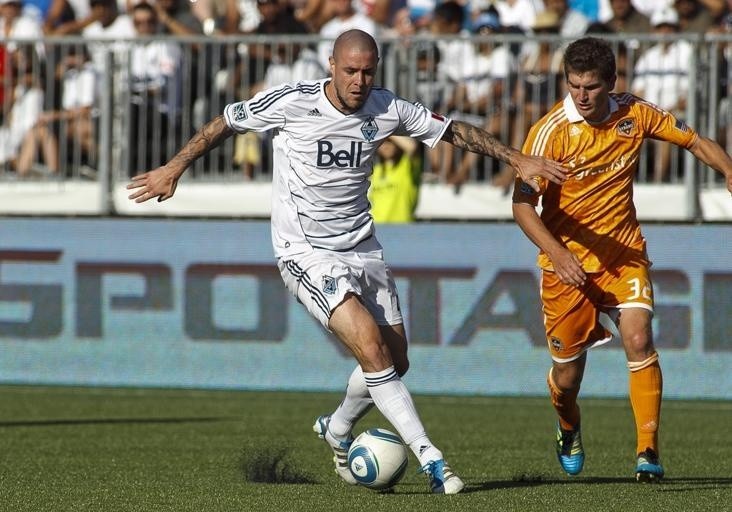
[533,12,563,32]
[472,14,502,32]
[651,5,680,28]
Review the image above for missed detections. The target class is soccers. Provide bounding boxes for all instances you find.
[347,428,408,489]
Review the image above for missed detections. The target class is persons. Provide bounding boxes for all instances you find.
[1,1,732,227]
[510,34,732,483]
[123,28,567,495]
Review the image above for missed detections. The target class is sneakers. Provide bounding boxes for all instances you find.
[416,458,464,495]
[557,419,583,474]
[635,446,663,483]
[312,413,359,485]
